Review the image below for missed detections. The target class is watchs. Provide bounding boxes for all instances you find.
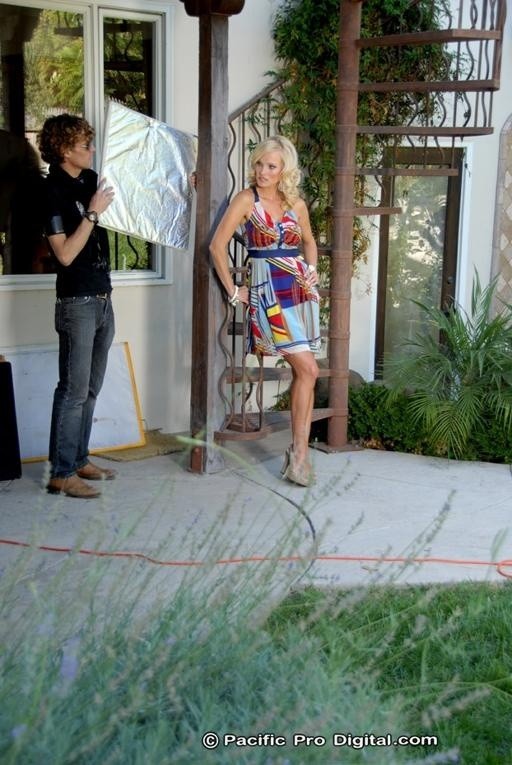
[84,211,99,224]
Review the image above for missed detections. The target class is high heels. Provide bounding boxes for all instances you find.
[280,442,314,487]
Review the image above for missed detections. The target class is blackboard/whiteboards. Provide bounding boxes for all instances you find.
[0,339,146,462]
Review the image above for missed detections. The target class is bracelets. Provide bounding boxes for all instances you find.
[308,264,316,272]
[228,284,239,307]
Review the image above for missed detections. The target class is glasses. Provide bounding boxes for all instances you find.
[67,142,91,150]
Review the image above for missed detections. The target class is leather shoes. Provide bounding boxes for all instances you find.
[77,461,118,480]
[46,476,100,497]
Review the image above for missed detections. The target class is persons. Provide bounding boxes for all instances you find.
[209,135,321,487]
[40,114,196,499]
